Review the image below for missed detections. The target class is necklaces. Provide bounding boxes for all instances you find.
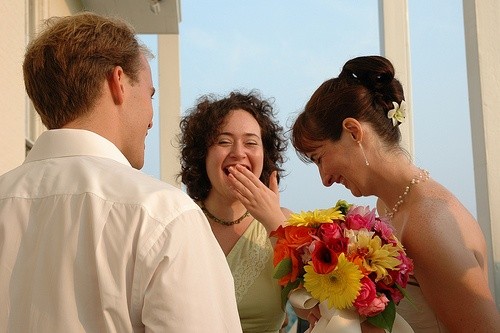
[384,167,430,224]
[200,199,250,227]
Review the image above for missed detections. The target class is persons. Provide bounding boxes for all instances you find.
[290,55,500,333]
[0,11,243,333]
[170,92,298,333]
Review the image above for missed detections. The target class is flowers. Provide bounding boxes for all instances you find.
[388,101,408,127]
[269,199,419,333]
